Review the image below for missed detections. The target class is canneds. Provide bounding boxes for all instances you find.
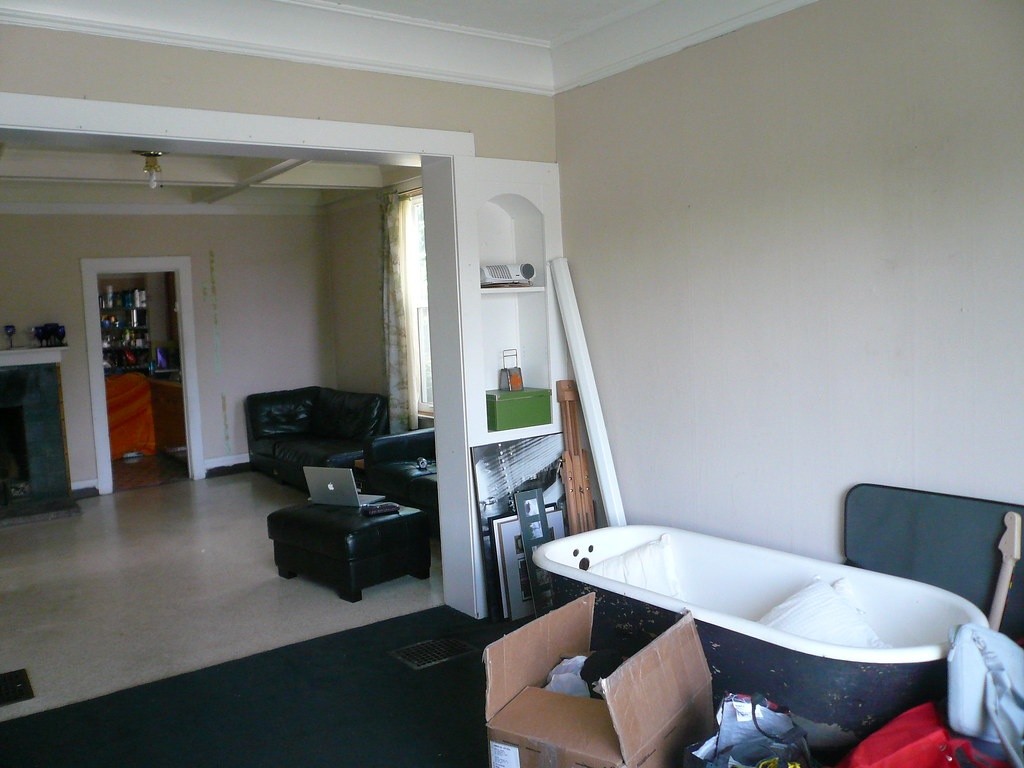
[416,456,427,469]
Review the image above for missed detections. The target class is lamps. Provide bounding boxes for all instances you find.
[133,150,172,190]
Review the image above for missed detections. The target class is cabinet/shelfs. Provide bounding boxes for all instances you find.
[98,289,153,377]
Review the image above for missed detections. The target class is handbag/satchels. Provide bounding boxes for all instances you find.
[680,692,813,768]
[836,702,1009,768]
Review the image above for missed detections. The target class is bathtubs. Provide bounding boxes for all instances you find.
[529,519,993,761]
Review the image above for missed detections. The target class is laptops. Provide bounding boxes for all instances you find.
[303,466,386,507]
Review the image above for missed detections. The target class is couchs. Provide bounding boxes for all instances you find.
[246,386,390,493]
[361,427,441,539]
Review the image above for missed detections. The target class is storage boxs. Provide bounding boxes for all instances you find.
[486,385,552,431]
[480,592,716,768]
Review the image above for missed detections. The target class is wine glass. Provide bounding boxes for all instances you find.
[34,321,65,348]
[5,323,15,349]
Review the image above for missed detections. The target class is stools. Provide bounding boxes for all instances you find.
[266,503,431,603]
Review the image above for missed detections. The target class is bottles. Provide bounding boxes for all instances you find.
[417,457,426,468]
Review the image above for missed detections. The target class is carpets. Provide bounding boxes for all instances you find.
[0,604,538,768]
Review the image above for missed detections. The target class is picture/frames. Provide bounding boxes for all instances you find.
[469,431,569,622]
